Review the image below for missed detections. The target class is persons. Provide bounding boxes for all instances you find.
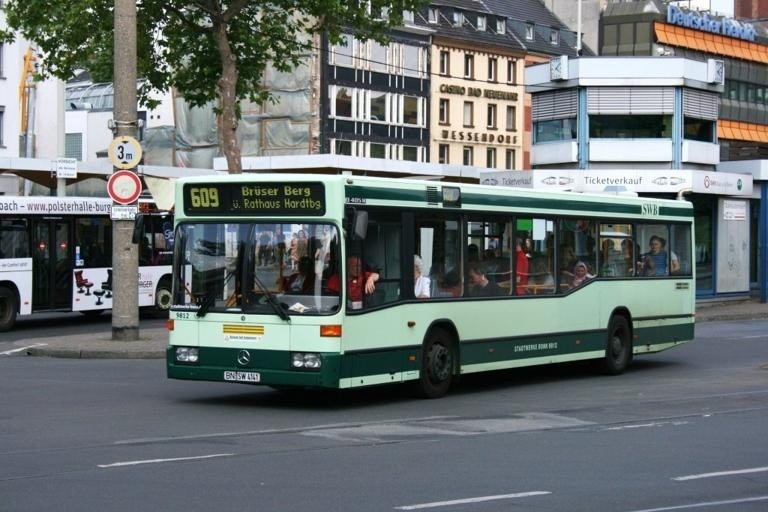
[293,226,381,313]
[396,229,681,302]
[253,227,307,270]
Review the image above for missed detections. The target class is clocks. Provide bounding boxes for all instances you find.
[549,54,569,81]
[706,58,726,87]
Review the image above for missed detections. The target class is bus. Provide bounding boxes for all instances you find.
[0,195,240,330]
[164,174,696,398]
